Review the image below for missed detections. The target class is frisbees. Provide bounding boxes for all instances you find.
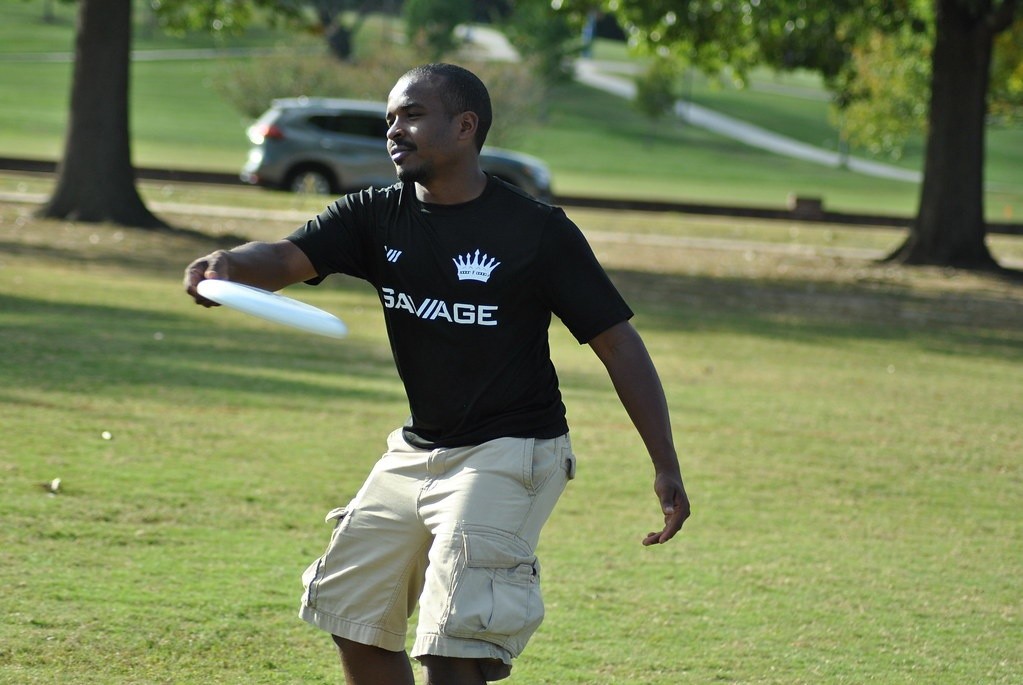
[194,278,349,342]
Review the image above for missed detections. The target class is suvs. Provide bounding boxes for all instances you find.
[241,96,555,204]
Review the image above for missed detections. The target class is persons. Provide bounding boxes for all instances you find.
[184,61,690,685]
